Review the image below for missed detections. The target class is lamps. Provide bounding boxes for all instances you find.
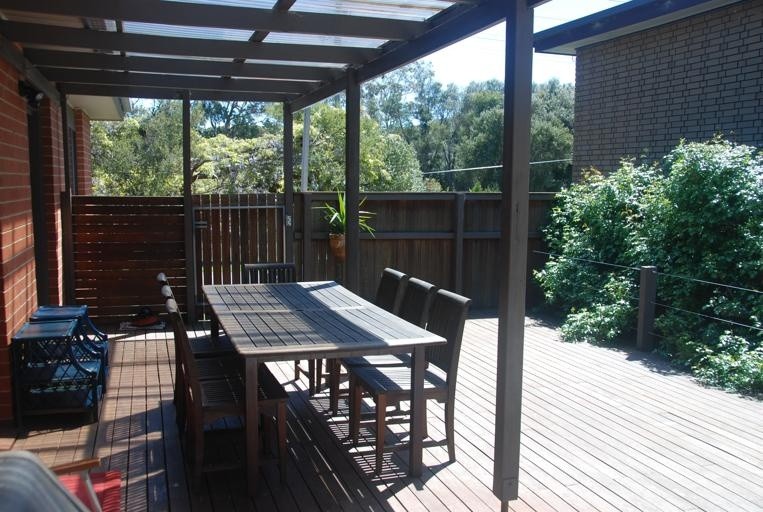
[19,89,40,108]
[19,79,45,101]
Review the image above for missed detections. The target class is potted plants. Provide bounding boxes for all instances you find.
[311,186,377,264]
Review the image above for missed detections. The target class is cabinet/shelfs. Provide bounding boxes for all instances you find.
[11,319,106,428]
[27,303,110,394]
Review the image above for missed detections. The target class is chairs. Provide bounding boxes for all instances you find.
[349,287,475,476]
[317,266,410,409]
[48,458,122,512]
[157,272,288,495]
[330,276,440,437]
[242,262,316,397]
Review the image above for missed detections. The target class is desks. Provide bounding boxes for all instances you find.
[202,281,451,496]
